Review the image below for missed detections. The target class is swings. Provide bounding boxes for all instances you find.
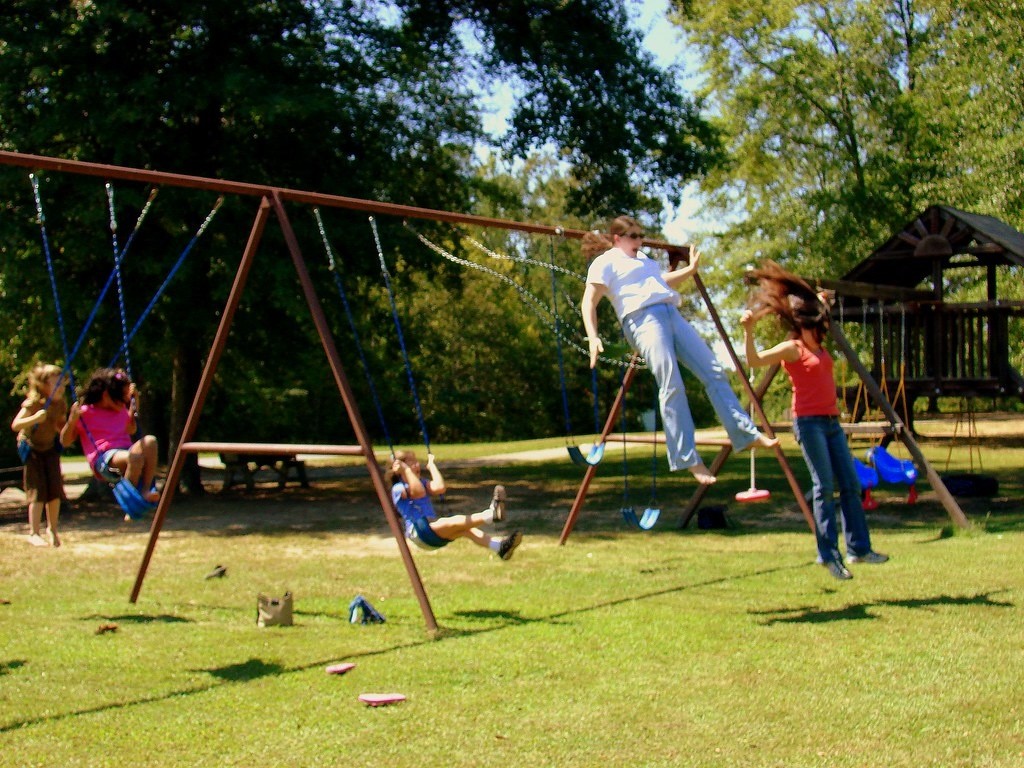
[312,206,457,550]
[838,295,880,511]
[17,188,226,468]
[401,218,655,374]
[618,324,662,531]
[545,231,606,468]
[864,308,920,485]
[27,169,160,523]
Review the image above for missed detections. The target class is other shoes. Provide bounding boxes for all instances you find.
[822,559,853,579]
[358,693,406,708]
[847,550,889,563]
[499,530,521,559]
[325,662,356,675]
[489,485,506,523]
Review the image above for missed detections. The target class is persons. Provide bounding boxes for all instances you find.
[385,450,523,561]
[11,365,68,547]
[581,214,780,485]
[737,258,890,580]
[60,368,160,523]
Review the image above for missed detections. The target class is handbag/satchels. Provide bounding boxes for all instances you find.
[256,591,293,628]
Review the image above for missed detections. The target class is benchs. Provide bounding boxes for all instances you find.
[218,453,311,496]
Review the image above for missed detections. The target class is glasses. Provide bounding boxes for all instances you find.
[623,232,645,239]
